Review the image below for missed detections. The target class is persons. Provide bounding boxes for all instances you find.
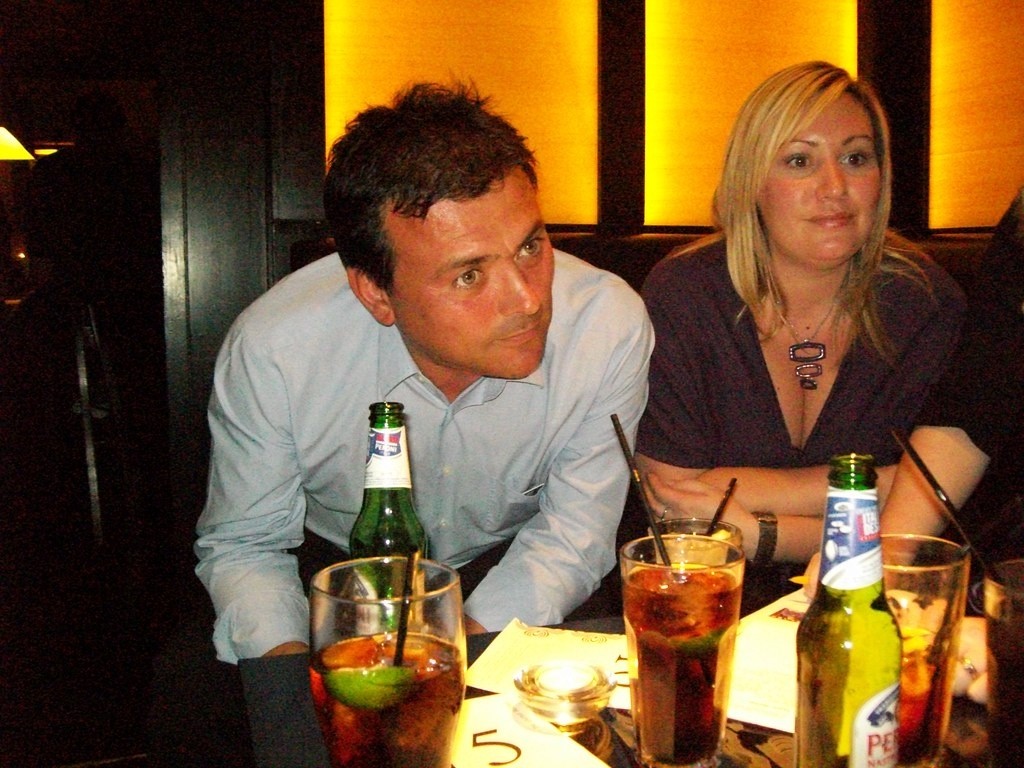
[633,60,1024,601]
[192,75,656,665]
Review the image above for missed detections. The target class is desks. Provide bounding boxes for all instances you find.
[237,617,993,768]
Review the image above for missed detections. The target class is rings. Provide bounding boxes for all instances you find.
[961,658,979,680]
[661,509,667,520]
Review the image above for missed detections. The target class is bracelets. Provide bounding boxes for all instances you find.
[747,511,778,581]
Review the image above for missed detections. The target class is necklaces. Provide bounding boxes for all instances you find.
[764,258,854,390]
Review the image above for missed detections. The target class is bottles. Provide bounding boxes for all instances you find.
[792,452,902,768]
[349,402,433,634]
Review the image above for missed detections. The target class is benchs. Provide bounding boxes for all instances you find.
[289,231,996,298]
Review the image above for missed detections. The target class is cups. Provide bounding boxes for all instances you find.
[864,533,972,768]
[983,557,1024,768]
[620,533,746,768]
[307,555,466,768]
[647,518,744,571]
